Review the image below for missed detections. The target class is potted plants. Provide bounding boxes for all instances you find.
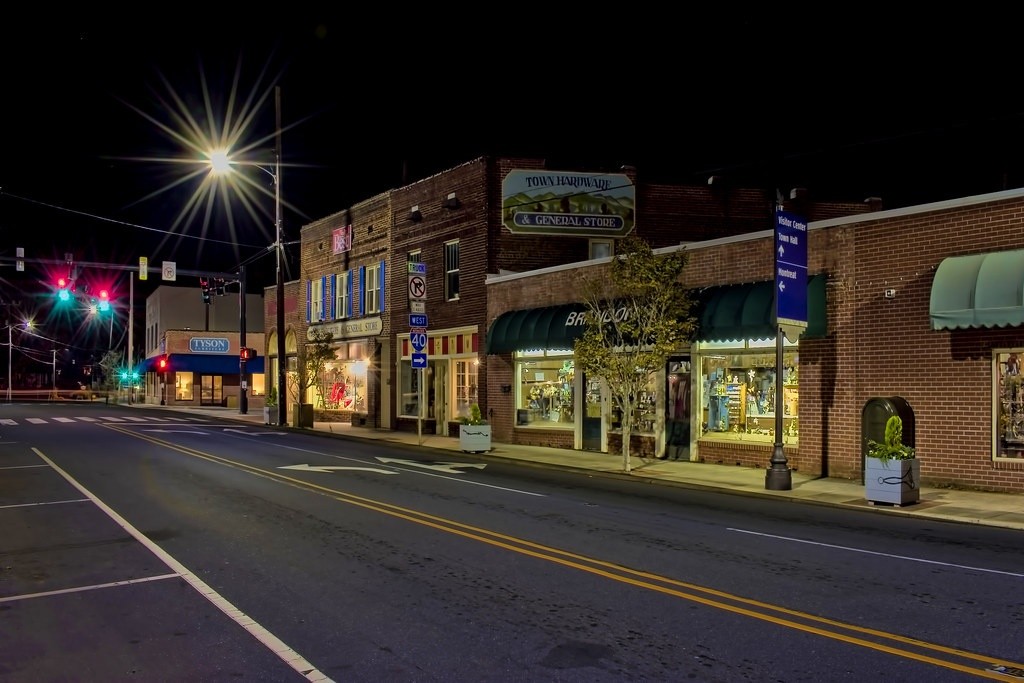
[264,388,277,426]
[455,403,492,455]
[865,415,920,508]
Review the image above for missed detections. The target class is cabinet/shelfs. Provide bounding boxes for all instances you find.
[722,382,747,432]
[518,409,534,425]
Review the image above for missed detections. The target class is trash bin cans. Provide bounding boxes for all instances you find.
[861,397,914,484]
[294,404,313,429]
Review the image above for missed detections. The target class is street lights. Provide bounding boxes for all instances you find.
[52,348,70,401]
[208,86,289,430]
[8,321,32,402]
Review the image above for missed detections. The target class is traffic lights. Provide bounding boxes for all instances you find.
[214,278,225,297]
[58,278,71,302]
[240,347,254,359]
[200,277,214,305]
[122,372,129,383]
[132,372,140,382]
[158,357,168,371]
[100,289,110,313]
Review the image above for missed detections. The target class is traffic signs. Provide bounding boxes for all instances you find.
[776,204,810,270]
[774,259,810,328]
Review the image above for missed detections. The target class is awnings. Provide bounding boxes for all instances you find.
[485,273,827,356]
[929,247,1024,331]
[164,353,265,374]
[138,355,167,375]
